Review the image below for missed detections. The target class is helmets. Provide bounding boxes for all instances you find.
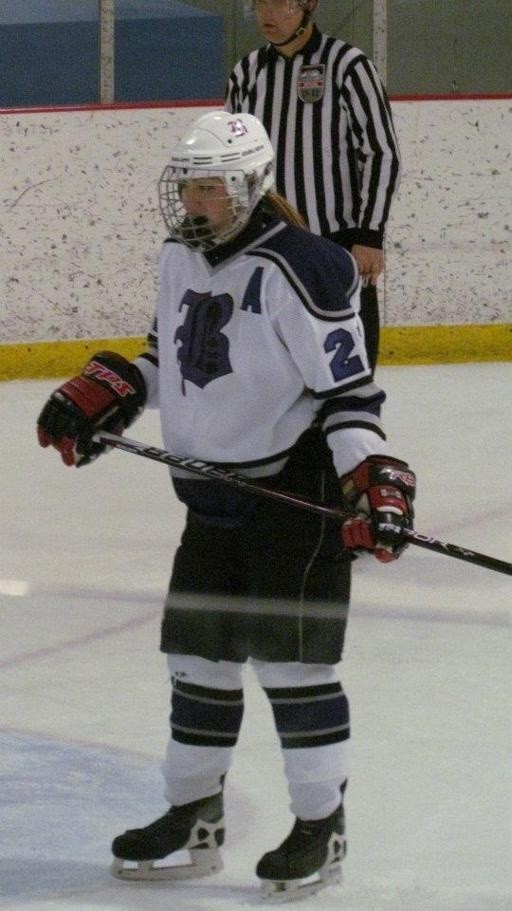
[156,112,277,252]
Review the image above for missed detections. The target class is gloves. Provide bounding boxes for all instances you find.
[338,453,416,567]
[37,350,148,471]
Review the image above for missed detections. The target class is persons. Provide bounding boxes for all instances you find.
[37,110,416,880]
[224,0,401,376]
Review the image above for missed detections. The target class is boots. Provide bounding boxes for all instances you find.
[257,778,350,881]
[113,769,227,858]
